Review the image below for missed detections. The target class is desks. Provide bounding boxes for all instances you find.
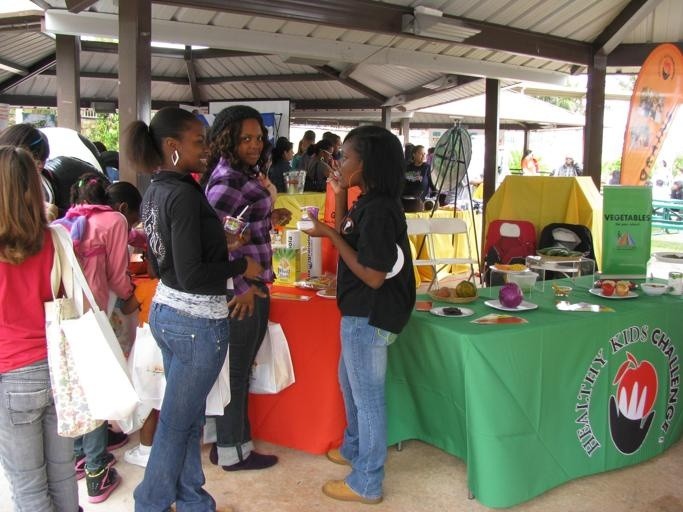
[383,274,683,509]
[129,273,348,454]
[405,209,480,281]
[274,191,325,273]
[483,176,603,269]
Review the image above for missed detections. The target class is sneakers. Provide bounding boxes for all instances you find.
[208,444,280,473]
[319,477,384,506]
[123,447,152,470]
[325,448,359,466]
[68,429,132,505]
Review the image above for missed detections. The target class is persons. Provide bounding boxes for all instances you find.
[121,106,267,510]
[545,154,584,177]
[260,127,343,191]
[202,104,294,470]
[530,152,539,175]
[608,156,683,201]
[37,125,147,452]
[518,150,536,175]
[0,120,48,176]
[36,179,143,504]
[297,124,418,505]
[400,140,437,201]
[0,144,81,510]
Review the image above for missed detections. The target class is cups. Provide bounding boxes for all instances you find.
[299,206,319,222]
[222,216,244,237]
[284,229,302,249]
[282,170,307,194]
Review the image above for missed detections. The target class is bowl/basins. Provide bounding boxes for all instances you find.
[641,282,668,296]
[550,285,572,298]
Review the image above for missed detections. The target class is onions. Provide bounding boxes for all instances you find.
[499,283,523,308]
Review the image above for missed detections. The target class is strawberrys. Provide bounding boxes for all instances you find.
[602,283,613,296]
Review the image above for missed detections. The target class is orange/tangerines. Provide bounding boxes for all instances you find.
[455,279,477,296]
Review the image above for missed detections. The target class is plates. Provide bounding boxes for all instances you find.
[426,288,479,303]
[588,287,640,300]
[535,250,584,261]
[484,298,539,312]
[429,306,475,318]
[302,277,329,289]
[651,250,683,263]
[488,265,529,274]
[315,289,337,300]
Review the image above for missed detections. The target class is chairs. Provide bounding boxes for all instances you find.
[430,219,478,289]
[484,221,535,288]
[539,223,597,280]
[405,218,436,290]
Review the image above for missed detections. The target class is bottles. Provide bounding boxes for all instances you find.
[667,271,683,296]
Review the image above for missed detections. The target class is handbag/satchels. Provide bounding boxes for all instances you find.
[127,321,234,419]
[244,318,299,396]
[36,290,141,441]
